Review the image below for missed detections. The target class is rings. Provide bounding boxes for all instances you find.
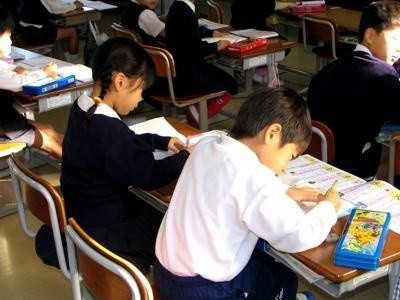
[326,179,338,197]
[185,138,189,148]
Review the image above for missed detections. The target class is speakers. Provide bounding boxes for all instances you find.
[64,50,70,63]
[69,53,85,65]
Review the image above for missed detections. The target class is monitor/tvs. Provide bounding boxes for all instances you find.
[286,0,331,13]
[267,244,324,285]
[15,63,40,75]
[198,17,279,45]
[81,0,119,11]
[127,115,188,160]
[282,153,399,234]
[23,55,93,82]
[288,178,355,221]
[9,52,25,61]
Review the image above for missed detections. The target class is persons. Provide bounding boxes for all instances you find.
[59,36,197,287]
[121,0,166,41]
[9,0,86,46]
[153,84,341,300]
[166,1,239,130]
[230,0,292,86]
[1,6,66,160]
[308,0,400,181]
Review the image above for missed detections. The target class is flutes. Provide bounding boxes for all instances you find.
[333,208,392,271]
[22,73,76,96]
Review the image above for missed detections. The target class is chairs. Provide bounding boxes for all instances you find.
[186,103,200,128]
[253,67,286,87]
[297,291,315,300]
[1,4,400,300]
